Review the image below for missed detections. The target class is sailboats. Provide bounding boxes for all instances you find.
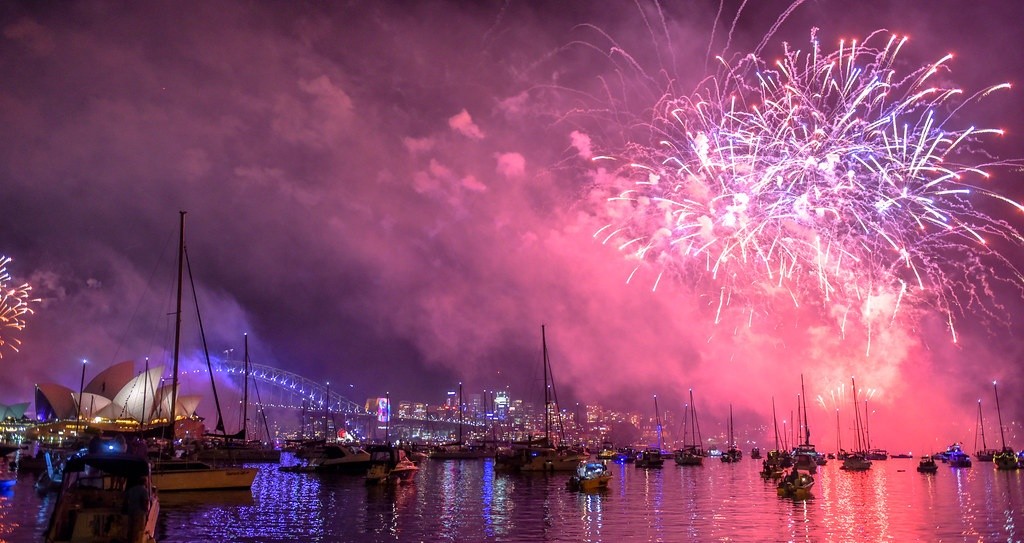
[972,381,1024,470]
[141,212,261,491]
[675,391,742,468]
[467,325,668,471]
[279,383,467,480]
[750,374,886,478]
[200,333,281,461]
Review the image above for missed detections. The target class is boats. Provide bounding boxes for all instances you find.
[43,434,160,543]
[776,470,816,496]
[932,446,972,469]
[6,441,85,491]
[890,454,913,458]
[568,457,613,492]
[917,459,938,472]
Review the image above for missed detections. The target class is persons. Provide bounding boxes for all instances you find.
[762,460,769,471]
[399,439,403,449]
[126,477,149,538]
[405,442,411,455]
[388,441,391,450]
[791,468,798,481]
